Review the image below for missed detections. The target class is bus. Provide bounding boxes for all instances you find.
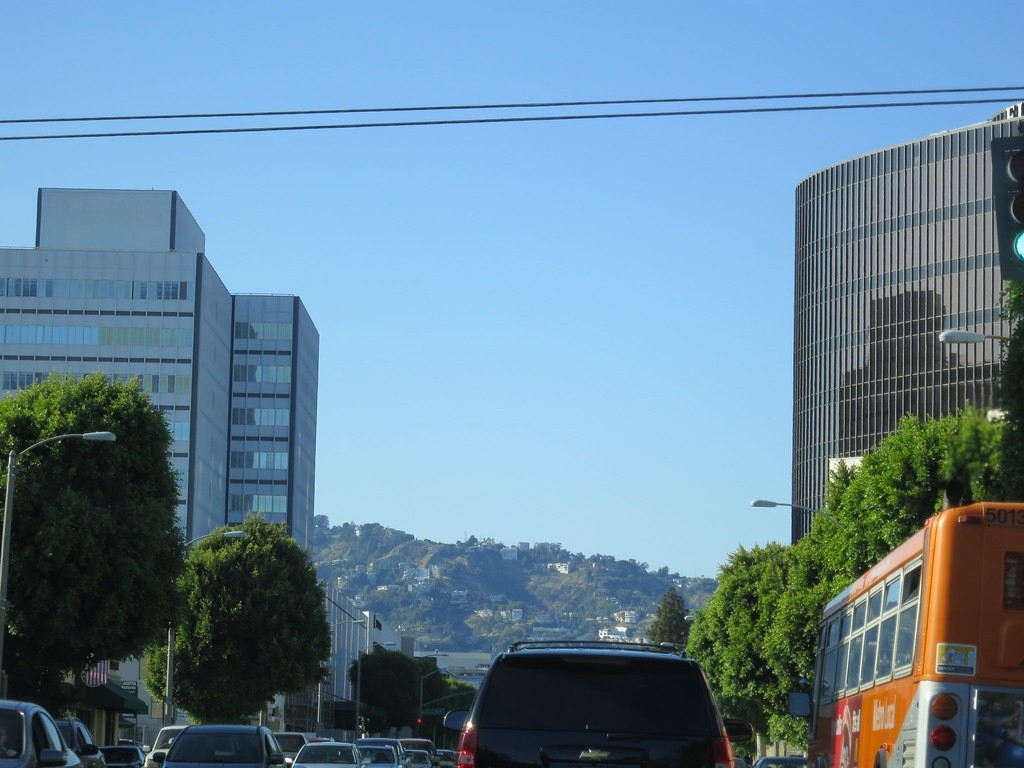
[787,499,1024,768]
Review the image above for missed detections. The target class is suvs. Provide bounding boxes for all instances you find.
[442,639,755,767]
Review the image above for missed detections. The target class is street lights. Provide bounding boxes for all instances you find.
[161,531,248,729]
[0,432,117,683]
[312,620,367,738]
[418,665,465,738]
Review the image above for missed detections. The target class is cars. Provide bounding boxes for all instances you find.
[0,696,85,768]
[142,721,457,768]
[756,754,807,768]
[97,742,146,768]
[53,717,107,768]
